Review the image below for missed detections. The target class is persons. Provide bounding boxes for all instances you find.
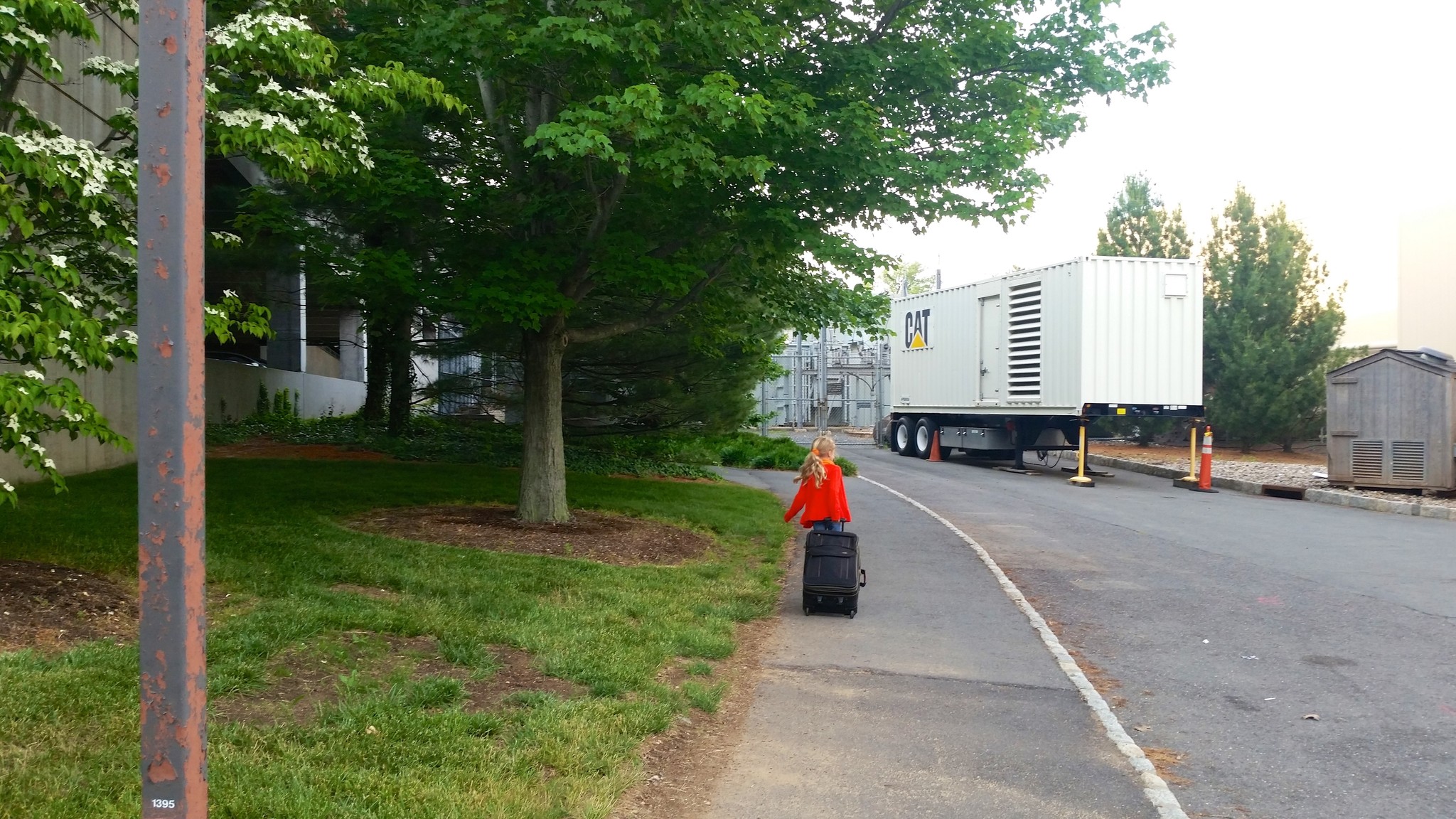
[783,435,852,532]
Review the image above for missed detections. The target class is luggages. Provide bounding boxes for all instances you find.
[802,516,866,618]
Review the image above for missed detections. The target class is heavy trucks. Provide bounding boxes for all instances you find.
[889,255,1205,463]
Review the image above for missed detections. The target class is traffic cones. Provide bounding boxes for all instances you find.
[1189,425,1219,493]
[926,430,944,462]
